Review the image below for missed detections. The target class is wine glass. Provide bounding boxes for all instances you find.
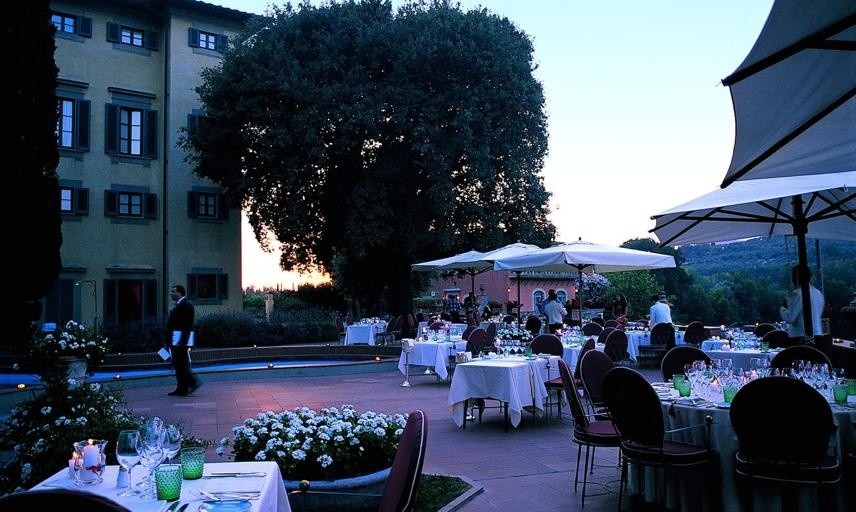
[563,329,584,345]
[116,417,182,500]
[624,321,644,331]
[360,317,380,325]
[494,340,526,360]
[731,338,763,351]
[420,326,463,342]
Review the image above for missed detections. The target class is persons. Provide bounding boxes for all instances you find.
[162,284,201,397]
[424,290,677,365]
[778,263,825,345]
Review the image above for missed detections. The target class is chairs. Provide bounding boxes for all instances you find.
[0,486,132,512]
[286,409,428,512]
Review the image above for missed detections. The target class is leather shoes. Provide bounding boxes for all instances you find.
[169,390,187,396]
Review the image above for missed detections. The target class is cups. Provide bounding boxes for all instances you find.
[180,447,204,480]
[762,343,769,351]
[673,358,856,408]
[645,328,648,331]
[525,350,532,357]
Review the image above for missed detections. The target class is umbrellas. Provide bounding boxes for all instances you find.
[411,248,493,325]
[491,236,677,329]
[647,170,855,341]
[719,0,856,188]
[479,241,546,337]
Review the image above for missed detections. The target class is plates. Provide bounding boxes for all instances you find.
[199,499,252,512]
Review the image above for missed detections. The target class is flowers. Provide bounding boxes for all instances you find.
[8,318,110,372]
[1,375,212,492]
[214,402,410,475]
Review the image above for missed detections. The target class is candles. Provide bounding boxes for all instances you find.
[68,450,83,477]
[83,439,99,481]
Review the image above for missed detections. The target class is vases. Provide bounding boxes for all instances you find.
[282,468,391,506]
[37,354,89,393]
